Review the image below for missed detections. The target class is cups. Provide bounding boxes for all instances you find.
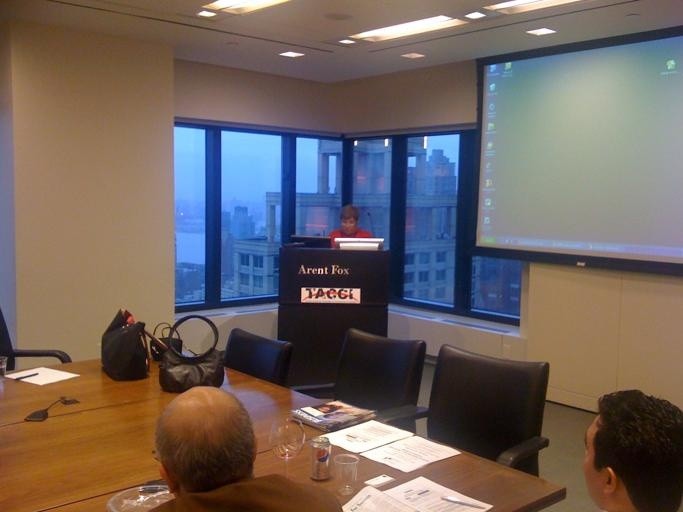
[333,453,359,497]
[0,356,7,376]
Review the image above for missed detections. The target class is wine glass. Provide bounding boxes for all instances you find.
[269,417,306,482]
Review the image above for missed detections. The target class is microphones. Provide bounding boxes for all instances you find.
[367,212,375,237]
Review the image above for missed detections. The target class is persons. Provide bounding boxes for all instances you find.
[144,385,344,511]
[583,387,683,511]
[327,205,372,247]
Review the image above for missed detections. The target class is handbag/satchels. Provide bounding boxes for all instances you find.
[159,315,227,392]
[98,308,151,382]
[150,323,184,361]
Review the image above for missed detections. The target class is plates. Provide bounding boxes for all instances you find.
[106,485,175,512]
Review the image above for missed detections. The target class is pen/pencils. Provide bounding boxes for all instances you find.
[441,496,486,510]
[16,373,39,380]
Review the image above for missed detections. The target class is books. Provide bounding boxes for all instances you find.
[290,398,376,433]
[342,476,493,511]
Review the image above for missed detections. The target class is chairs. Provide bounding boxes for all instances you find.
[223,327,294,385]
[290,327,427,419]
[371,343,550,478]
[0,305,72,372]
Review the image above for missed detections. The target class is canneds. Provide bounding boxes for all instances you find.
[310,435,332,480]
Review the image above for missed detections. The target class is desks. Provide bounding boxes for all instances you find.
[0,355,255,427]
[0,377,377,512]
[37,421,567,512]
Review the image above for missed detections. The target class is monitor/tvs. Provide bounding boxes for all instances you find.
[290,235,331,248]
[334,237,384,251]
